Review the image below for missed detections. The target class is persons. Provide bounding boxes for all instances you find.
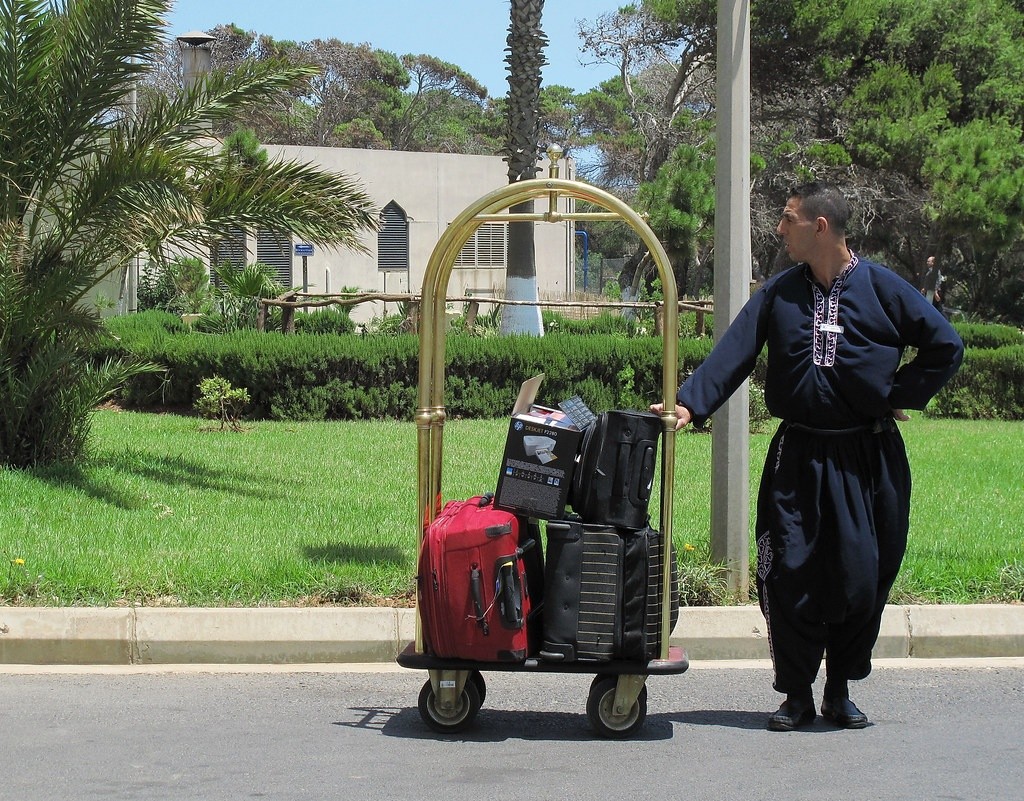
[647,177,966,731]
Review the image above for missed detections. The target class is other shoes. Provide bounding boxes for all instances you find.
[768,696,868,733]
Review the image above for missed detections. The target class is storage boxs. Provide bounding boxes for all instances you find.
[492,372,595,520]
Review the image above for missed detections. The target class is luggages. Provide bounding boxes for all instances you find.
[414,409,678,662]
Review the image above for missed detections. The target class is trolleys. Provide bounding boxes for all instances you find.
[395,144,686,736]
